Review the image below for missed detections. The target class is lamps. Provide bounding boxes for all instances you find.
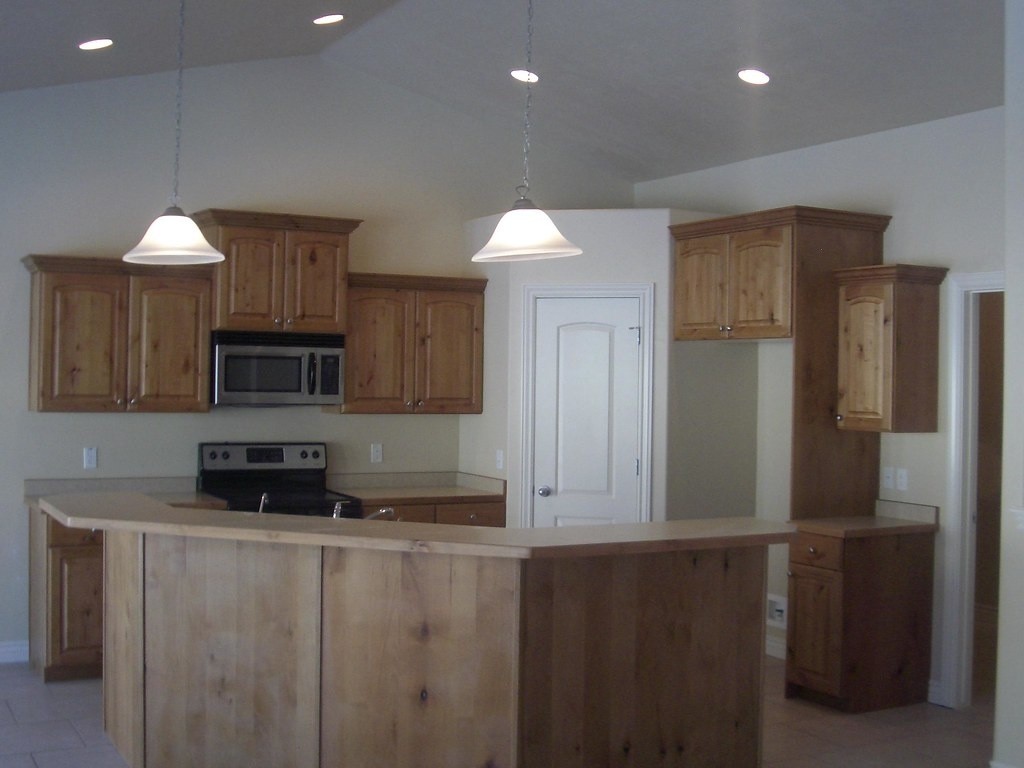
[122,0,224,265]
[471,0,582,262]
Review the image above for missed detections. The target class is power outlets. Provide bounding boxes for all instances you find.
[371,444,382,463]
[496,450,503,470]
[84,447,97,468]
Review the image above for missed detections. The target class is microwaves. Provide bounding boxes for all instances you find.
[210,336,346,408]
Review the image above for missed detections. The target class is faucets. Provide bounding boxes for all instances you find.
[258,492,269,513]
[362,505,395,520]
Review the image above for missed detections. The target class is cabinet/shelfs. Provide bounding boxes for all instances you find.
[783,515,938,713]
[667,205,893,342]
[28,508,102,684]
[20,254,214,413]
[321,272,488,415]
[832,264,950,434]
[186,208,365,336]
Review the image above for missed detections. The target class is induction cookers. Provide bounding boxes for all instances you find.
[195,440,362,508]
